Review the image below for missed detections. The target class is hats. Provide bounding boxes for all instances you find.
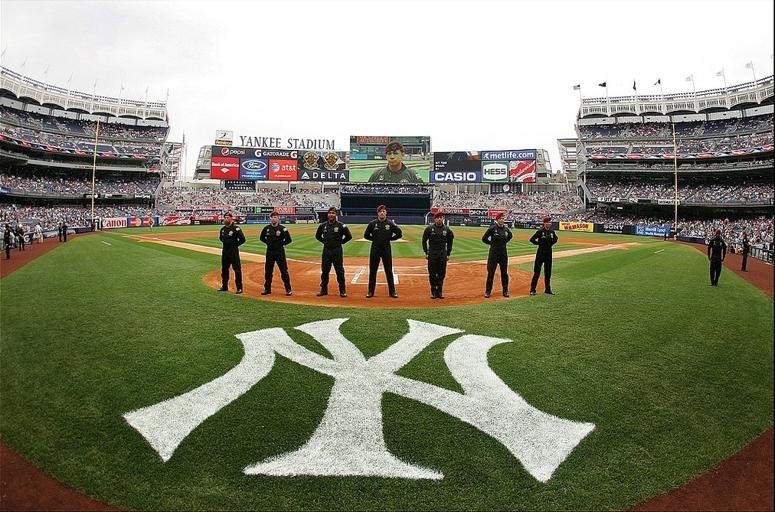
[384,140,403,155]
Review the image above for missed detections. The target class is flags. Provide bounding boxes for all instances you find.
[685,74,693,82]
[715,69,725,77]
[598,82,606,87]
[0,46,171,97]
[744,61,753,68]
[632,81,636,91]
[573,84,580,91]
[653,79,661,85]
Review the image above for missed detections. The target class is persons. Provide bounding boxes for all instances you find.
[529,217,559,296]
[315,207,353,297]
[740,232,753,272]
[421,211,454,300]
[0,102,774,262]
[481,212,513,299]
[364,205,403,299]
[706,227,728,289]
[260,211,292,297]
[215,211,247,294]
